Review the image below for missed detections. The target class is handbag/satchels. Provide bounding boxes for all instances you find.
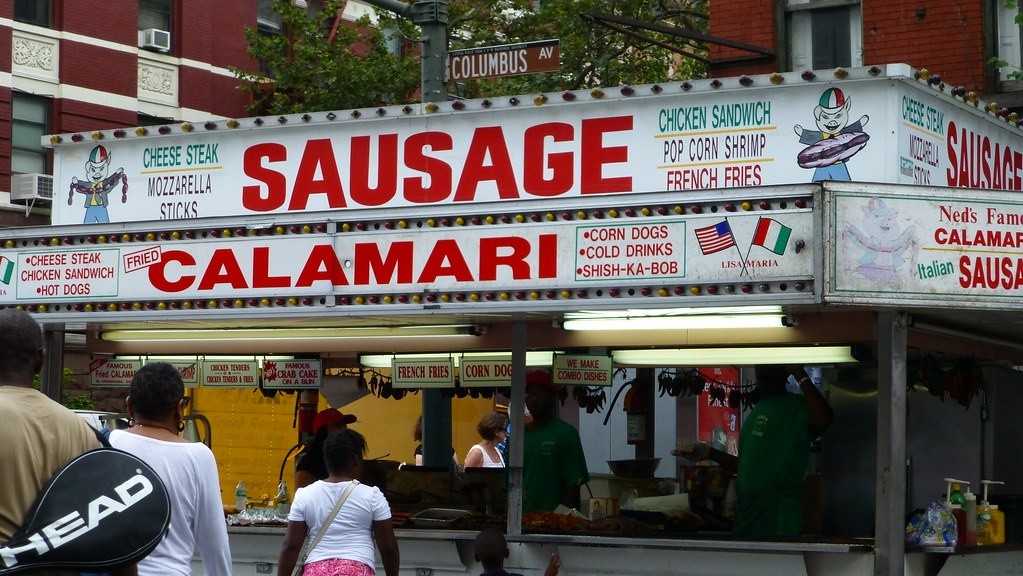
[453,458,463,473]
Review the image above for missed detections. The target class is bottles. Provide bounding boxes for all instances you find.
[977,500,992,545]
[963,485,977,546]
[951,505,966,545]
[949,484,967,508]
[987,505,1004,543]
[234,480,247,509]
[277,479,290,514]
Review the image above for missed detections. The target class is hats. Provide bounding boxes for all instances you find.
[313,408,357,432]
[526,371,563,395]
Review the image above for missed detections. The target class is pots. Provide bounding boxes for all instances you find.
[604,457,663,475]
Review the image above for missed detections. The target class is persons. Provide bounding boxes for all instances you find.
[100,361,233,576]
[411,415,461,470]
[681,365,835,542]
[276,428,399,576]
[464,412,507,469]
[474,527,559,576]
[0,307,103,545]
[295,408,357,487]
[500,400,534,468]
[502,382,590,516]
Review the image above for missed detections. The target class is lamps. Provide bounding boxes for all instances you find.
[98,309,864,368]
[908,316,1023,351]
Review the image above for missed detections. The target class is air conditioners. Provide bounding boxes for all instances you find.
[140,28,170,53]
[12,173,53,202]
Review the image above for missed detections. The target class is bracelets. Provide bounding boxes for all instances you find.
[799,377,810,386]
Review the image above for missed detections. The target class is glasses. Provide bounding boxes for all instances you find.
[173,396,191,408]
[494,427,507,432]
